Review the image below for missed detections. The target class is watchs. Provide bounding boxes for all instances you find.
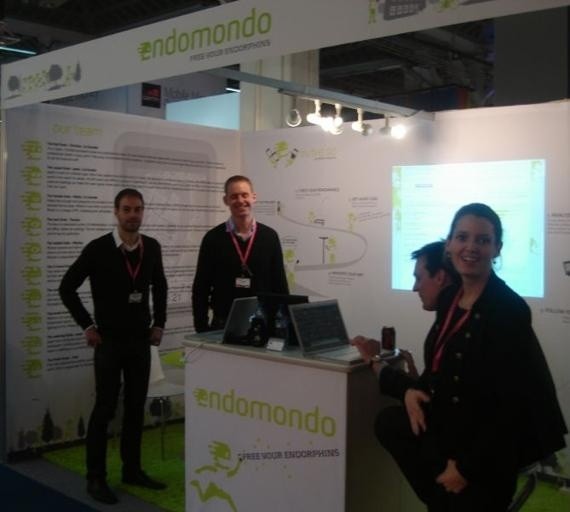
[367,354,384,370]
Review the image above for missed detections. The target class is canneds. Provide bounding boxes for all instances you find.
[382,325,395,350]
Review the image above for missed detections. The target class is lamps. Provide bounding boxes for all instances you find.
[283,93,417,141]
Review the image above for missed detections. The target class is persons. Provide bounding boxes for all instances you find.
[191,172,292,336]
[349,239,465,507]
[55,185,170,505]
[404,200,570,511]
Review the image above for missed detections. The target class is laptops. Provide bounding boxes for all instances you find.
[288,298,396,366]
[184,296,258,344]
[257,291,309,346]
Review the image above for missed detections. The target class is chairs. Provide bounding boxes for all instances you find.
[121,340,185,462]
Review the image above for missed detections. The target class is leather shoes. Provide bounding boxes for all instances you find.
[85,475,121,505]
[124,466,168,492]
